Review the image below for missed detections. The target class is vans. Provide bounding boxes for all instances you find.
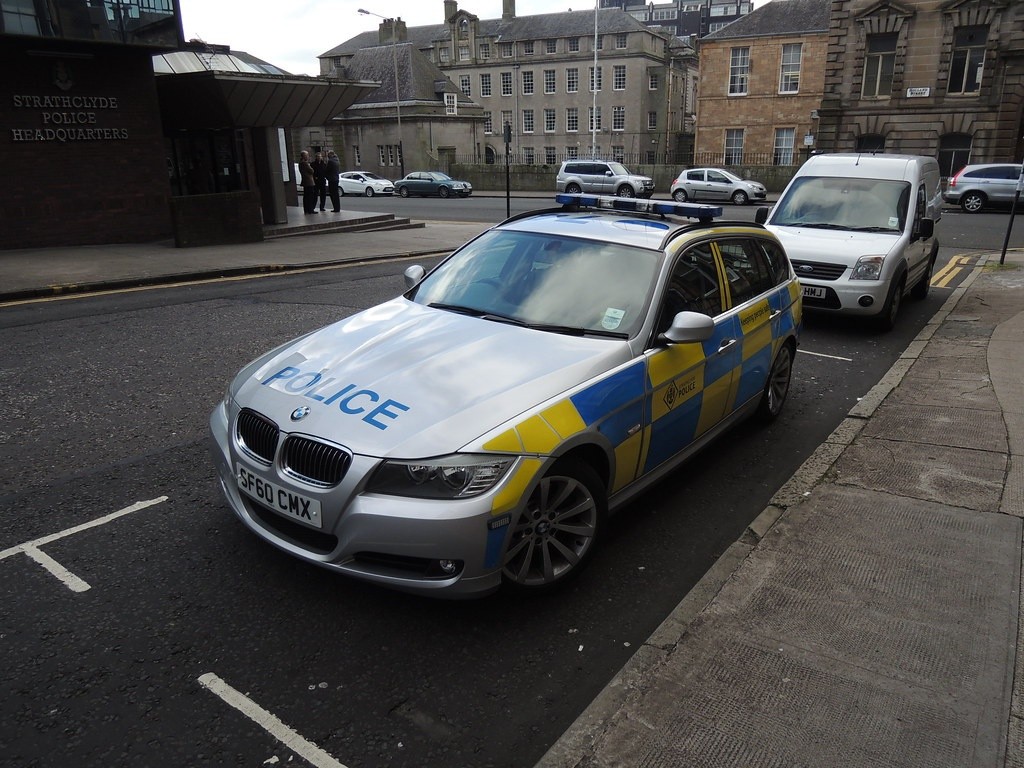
[754,152,943,333]
[293,162,330,197]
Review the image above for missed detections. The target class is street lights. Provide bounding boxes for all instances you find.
[356,8,405,179]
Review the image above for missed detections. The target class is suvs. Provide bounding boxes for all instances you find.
[556,158,656,199]
[944,163,1024,214]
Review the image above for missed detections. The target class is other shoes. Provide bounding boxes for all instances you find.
[320,208,326,212]
[331,209,340,212]
[304,211,318,215]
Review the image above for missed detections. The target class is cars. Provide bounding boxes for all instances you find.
[209,191,804,619]
[394,171,473,198]
[671,168,767,206]
[338,171,396,197]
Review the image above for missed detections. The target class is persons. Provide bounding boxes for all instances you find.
[299,150,340,215]
